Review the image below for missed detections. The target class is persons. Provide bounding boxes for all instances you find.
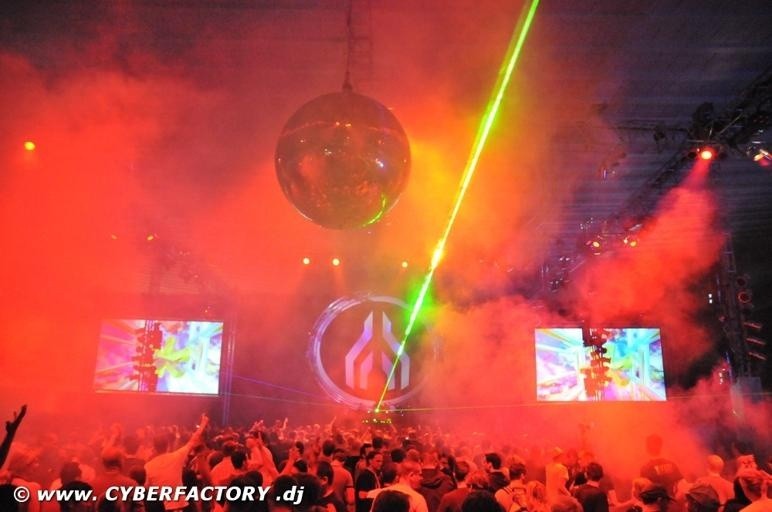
[0,402,772,512]
